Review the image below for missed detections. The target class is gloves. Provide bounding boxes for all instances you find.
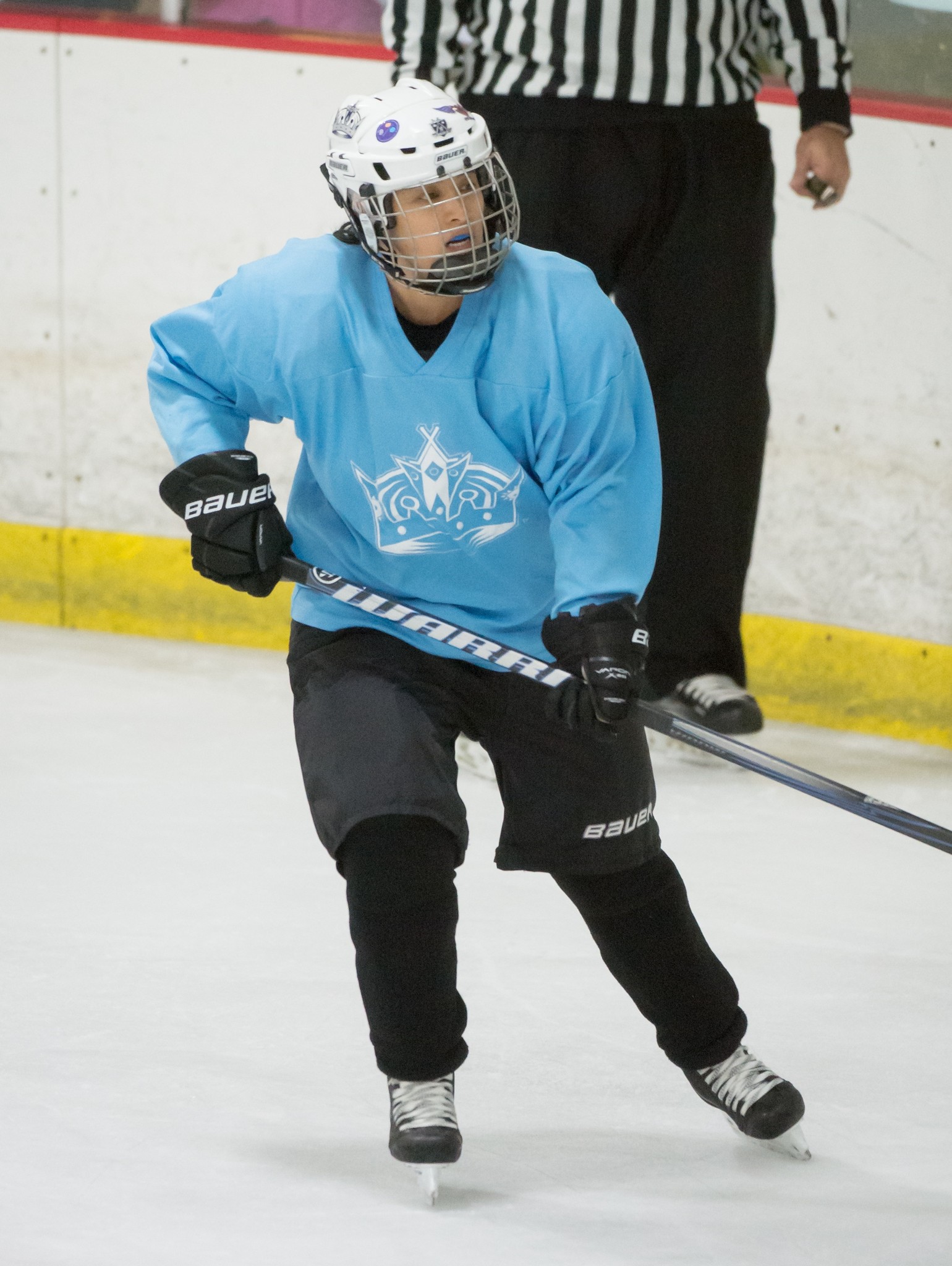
[159,450,294,597]
[541,602,653,733]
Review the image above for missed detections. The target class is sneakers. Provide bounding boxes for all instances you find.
[686,1045,816,1161]
[387,1072,462,1203]
[649,674,763,766]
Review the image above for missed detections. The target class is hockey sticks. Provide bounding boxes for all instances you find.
[279,553,952,858]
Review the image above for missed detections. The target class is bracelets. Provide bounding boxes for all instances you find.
[823,119,850,136]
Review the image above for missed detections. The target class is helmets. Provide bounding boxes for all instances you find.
[320,78,520,296]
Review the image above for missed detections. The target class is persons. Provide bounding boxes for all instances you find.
[146,85,816,1201]
[382,0,854,764]
